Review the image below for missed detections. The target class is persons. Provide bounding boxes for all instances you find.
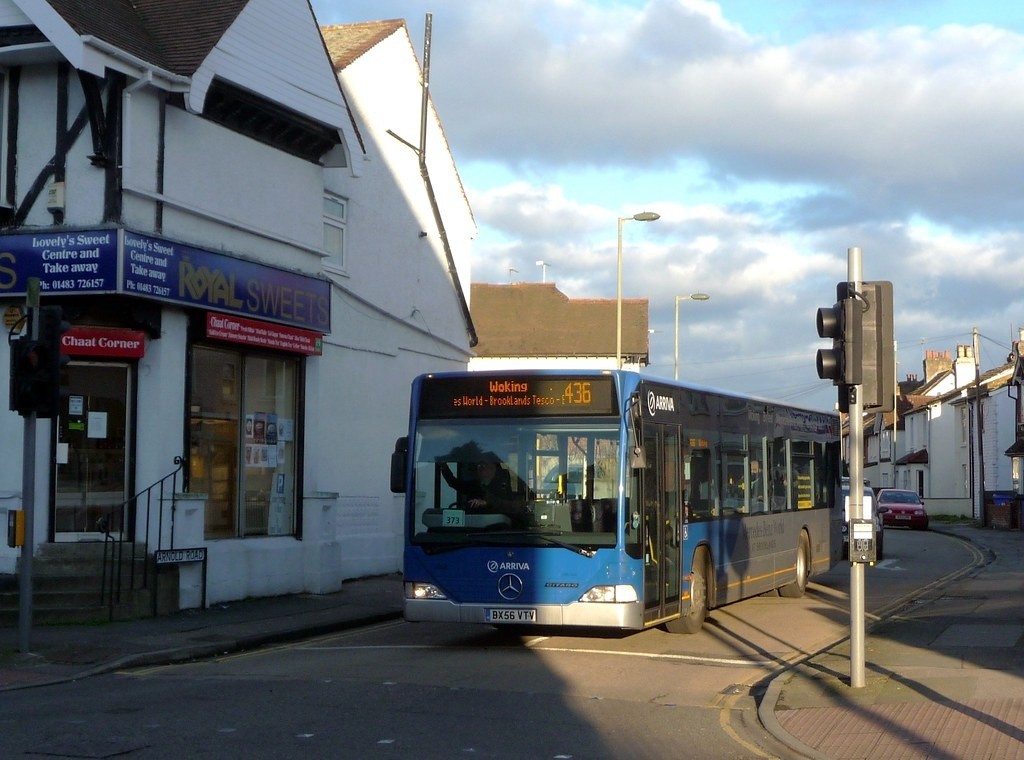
[441,452,511,532]
[740,459,763,498]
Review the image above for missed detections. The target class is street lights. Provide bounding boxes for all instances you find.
[675,294,709,380]
[617,213,660,370]
[834,402,843,461]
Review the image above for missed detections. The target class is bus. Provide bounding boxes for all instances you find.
[391,369,843,633]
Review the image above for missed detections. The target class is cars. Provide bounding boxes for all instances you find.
[540,464,605,499]
[876,489,929,530]
[818,486,889,560]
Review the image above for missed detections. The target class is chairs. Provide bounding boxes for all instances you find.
[723,496,786,514]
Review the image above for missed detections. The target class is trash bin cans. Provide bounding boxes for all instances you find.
[992,493,1014,506]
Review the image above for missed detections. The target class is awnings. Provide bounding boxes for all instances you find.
[891,449,928,466]
[1004,439,1024,457]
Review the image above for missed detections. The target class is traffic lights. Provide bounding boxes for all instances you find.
[9,336,49,419]
[27,305,70,418]
[816,299,862,386]
